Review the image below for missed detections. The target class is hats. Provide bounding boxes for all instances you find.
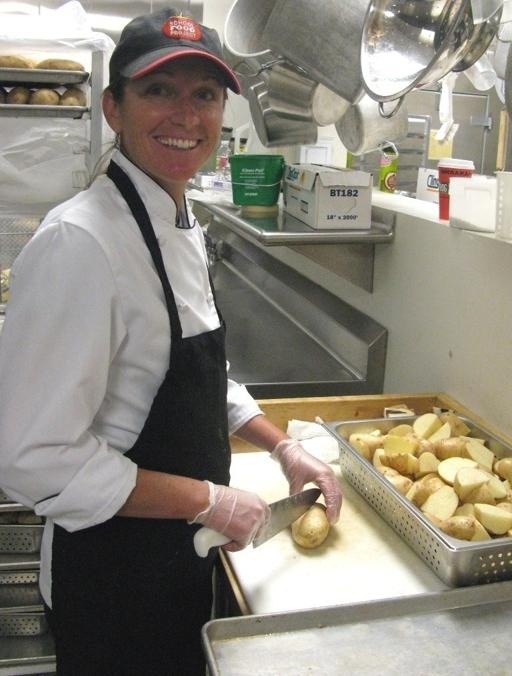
[111,5,241,96]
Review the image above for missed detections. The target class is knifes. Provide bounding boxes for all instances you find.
[192,489,323,558]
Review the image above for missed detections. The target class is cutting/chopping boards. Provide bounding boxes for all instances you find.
[224,448,450,617]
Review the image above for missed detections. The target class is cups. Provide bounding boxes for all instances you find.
[437,156,474,222]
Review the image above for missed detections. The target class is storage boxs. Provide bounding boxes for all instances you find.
[283,163,373,229]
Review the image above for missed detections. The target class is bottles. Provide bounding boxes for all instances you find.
[214,127,247,192]
[378,143,399,194]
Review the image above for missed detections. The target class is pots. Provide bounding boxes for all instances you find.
[219,0,502,154]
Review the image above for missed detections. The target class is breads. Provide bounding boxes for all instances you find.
[0,53,86,71]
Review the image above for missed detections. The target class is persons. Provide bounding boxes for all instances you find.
[0,5,345,674]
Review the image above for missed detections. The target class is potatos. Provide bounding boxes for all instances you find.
[346,413,512,544]
[289,500,330,549]
[0,86,87,107]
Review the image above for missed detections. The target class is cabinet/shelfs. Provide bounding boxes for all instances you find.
[207,392,512,615]
[3,54,103,673]
